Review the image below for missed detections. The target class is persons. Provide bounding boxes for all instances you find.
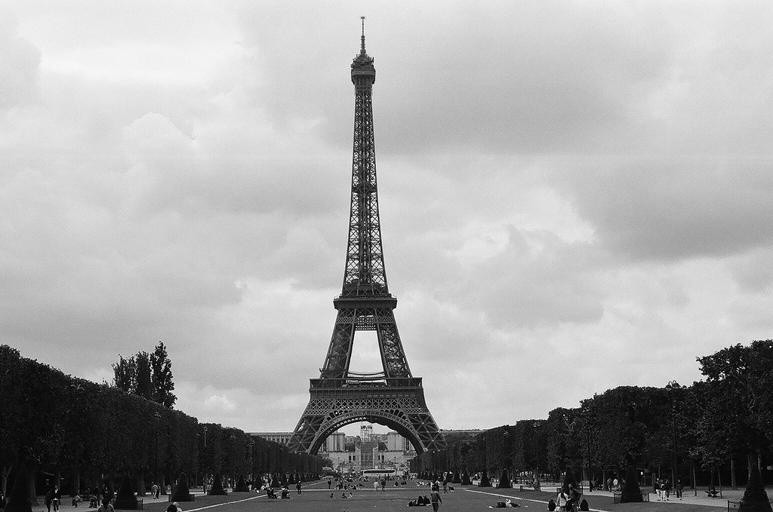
[339,474,357,500]
[335,479,340,489]
[612,476,619,490]
[654,477,683,501]
[415,480,428,488]
[372,477,408,494]
[244,479,253,492]
[565,501,575,512]
[69,483,118,511]
[407,494,430,506]
[296,480,302,495]
[328,492,334,504]
[606,476,612,491]
[327,478,331,488]
[430,487,442,511]
[166,504,177,512]
[556,487,569,512]
[49,484,61,512]
[580,499,589,512]
[151,481,160,499]
[266,486,291,500]
[44,484,51,512]
[442,477,448,494]
[547,497,555,511]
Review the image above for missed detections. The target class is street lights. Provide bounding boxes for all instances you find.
[203,426,207,493]
[665,380,681,495]
[581,407,593,492]
[533,422,541,475]
[154,412,159,483]
